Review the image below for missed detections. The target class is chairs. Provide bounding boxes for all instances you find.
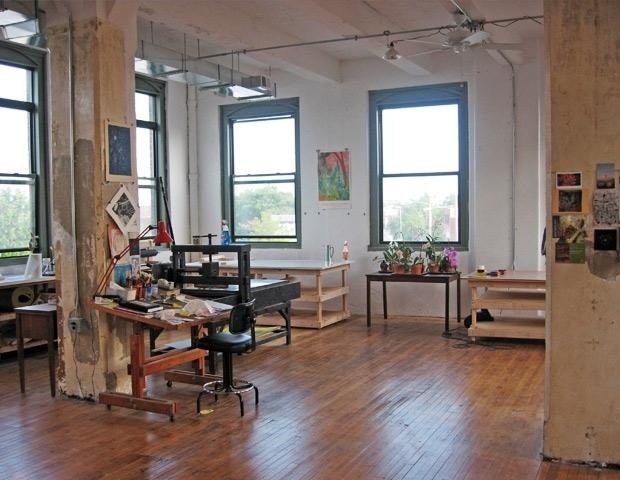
[196,296,259,415]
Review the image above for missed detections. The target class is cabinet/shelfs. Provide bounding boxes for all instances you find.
[186,258,352,330]
[0,273,55,354]
[459,268,546,342]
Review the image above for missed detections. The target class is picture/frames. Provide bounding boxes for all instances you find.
[105,183,139,235]
[104,117,137,182]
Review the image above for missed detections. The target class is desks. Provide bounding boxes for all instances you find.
[88,291,233,421]
[13,302,58,397]
[365,270,462,332]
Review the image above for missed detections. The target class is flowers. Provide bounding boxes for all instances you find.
[374,231,458,273]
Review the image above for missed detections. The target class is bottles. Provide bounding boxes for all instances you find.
[324,245,332,268]
[342,242,349,262]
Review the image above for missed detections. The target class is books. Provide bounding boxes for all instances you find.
[119,300,163,313]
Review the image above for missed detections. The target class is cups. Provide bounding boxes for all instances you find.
[42,258,51,274]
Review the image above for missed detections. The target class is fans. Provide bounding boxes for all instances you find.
[396,12,523,67]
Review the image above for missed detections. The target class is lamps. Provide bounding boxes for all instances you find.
[383,41,401,61]
[92,221,174,299]
